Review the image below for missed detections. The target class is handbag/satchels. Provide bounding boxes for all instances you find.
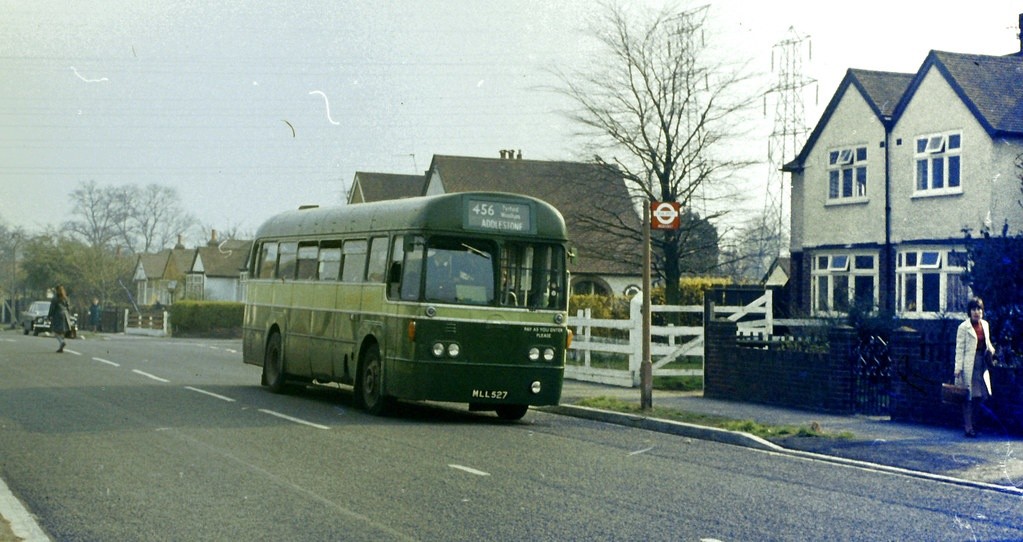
[940,379,970,406]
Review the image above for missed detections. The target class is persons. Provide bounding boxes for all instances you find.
[955,297,996,435]
[89,297,101,332]
[411,250,451,303]
[47,285,71,353]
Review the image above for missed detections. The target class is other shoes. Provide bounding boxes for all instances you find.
[56,342,65,353]
[965,428,976,437]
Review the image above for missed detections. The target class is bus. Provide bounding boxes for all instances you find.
[242,191,579,422]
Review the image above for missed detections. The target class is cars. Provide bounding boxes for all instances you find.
[19,301,78,338]
